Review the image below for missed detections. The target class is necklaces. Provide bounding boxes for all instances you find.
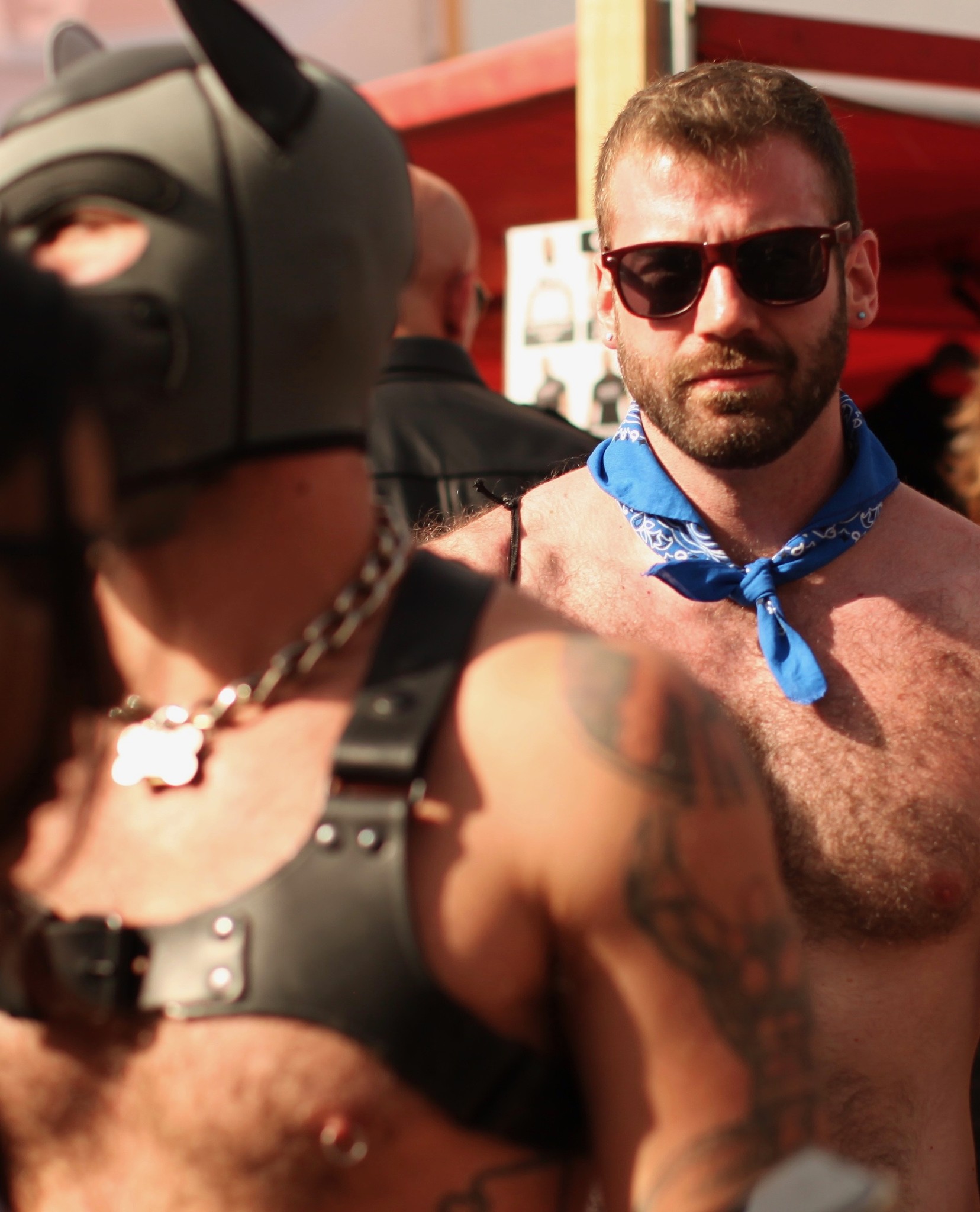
[110,510,417,785]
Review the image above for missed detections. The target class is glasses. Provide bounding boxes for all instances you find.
[601,222,851,320]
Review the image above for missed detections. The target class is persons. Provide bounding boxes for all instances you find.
[419,57,980,1212]
[0,0,978,1212]
